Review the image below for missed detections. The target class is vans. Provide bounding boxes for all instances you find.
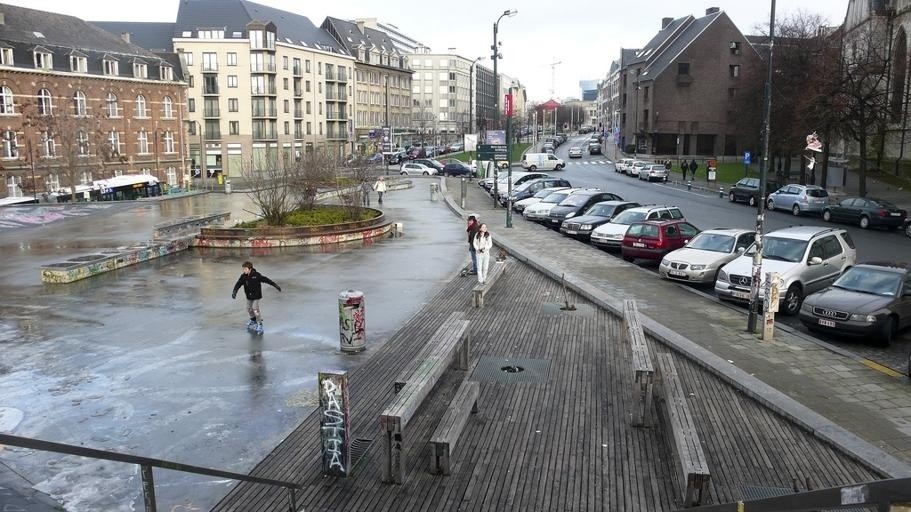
[521,152,566,172]
[728,176,784,207]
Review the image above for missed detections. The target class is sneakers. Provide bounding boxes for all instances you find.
[250,318,263,331]
[469,270,476,274]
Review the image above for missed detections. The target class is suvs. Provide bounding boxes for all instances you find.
[713,222,858,316]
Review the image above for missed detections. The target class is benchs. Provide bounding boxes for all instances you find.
[621,299,654,428]
[472,262,507,308]
[394,311,466,394]
[656,351,711,507]
[381,321,471,485]
[429,379,480,476]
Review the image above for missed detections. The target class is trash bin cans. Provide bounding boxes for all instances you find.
[339,290,366,353]
[430,183,440,202]
[225,181,231,194]
[706,168,717,183]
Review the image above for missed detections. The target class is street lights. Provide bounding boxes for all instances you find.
[489,9,519,124]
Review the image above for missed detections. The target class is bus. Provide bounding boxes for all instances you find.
[2,171,172,206]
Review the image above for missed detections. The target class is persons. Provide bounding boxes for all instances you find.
[232,261,281,332]
[681,160,689,180]
[655,159,672,175]
[374,176,387,203]
[359,176,374,206]
[690,158,698,180]
[466,216,480,275]
[473,223,493,287]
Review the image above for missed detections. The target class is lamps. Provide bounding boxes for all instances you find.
[655,112,660,123]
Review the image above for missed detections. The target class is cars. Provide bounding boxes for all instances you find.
[820,193,906,232]
[611,156,670,180]
[797,259,911,350]
[541,127,603,158]
[342,138,479,178]
[767,181,830,219]
[475,170,767,287]
